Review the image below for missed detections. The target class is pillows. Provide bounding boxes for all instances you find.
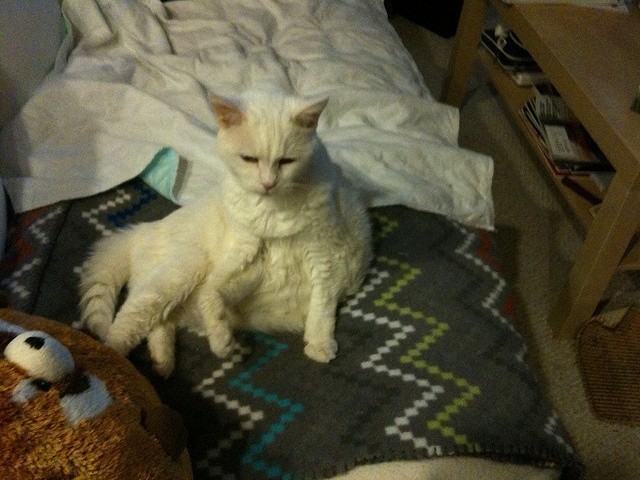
[2,176,589,477]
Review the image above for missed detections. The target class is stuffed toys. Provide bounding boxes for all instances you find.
[0,309,194,480]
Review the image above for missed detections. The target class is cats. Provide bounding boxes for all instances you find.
[75,87,376,381]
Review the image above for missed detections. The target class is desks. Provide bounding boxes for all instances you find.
[445,0,640,342]
